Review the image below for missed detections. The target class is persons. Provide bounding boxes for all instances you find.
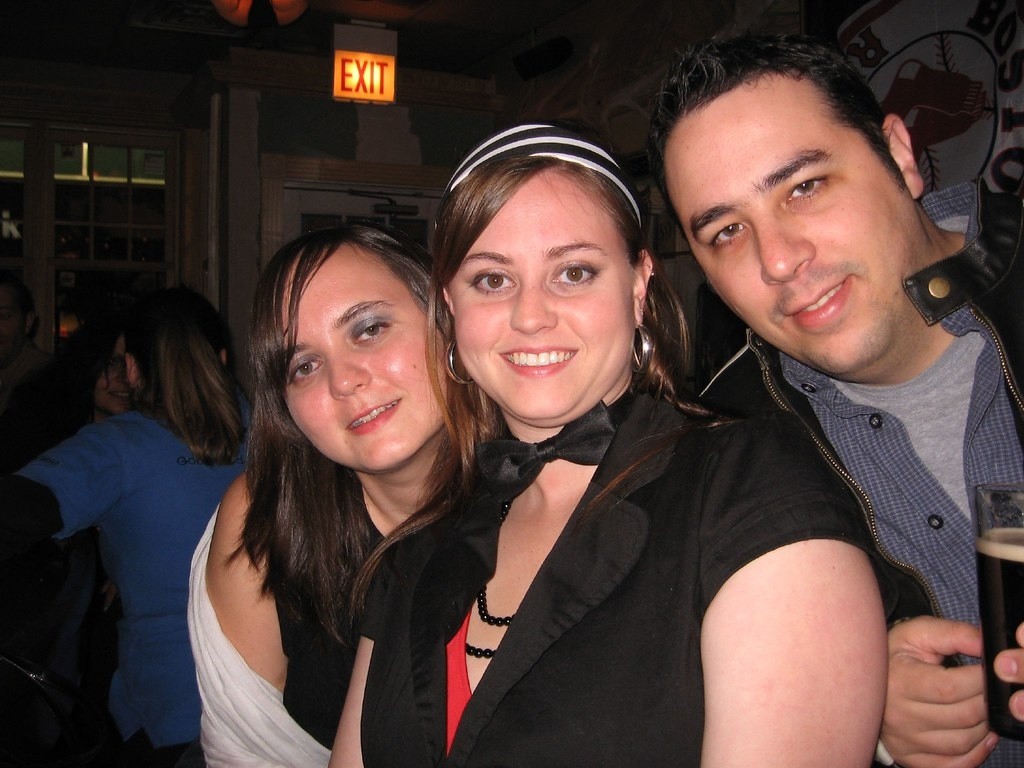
[188,215,453,767]
[0,265,253,768]
[324,123,890,768]
[648,33,1024,768]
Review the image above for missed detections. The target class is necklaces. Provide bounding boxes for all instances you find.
[461,498,521,658]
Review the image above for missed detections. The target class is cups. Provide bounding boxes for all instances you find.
[970,484,1024,741]
